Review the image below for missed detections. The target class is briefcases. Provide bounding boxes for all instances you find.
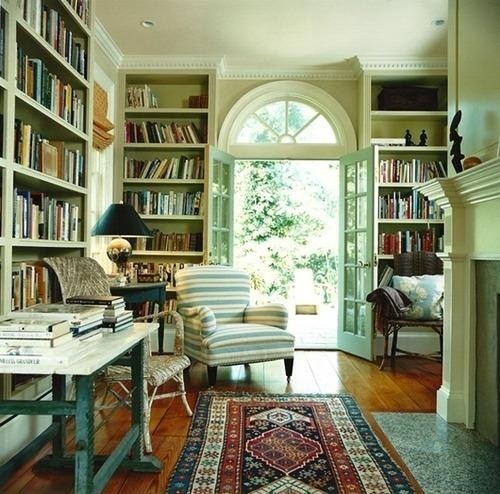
[377,86,438,110]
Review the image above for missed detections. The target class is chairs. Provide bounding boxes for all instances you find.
[40,256,194,454]
[376,251,443,372]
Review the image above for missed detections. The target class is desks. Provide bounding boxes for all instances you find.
[109,280,168,355]
[0,322,162,494]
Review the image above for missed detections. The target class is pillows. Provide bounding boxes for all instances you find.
[391,274,443,321]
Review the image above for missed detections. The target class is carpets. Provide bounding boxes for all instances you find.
[164,389,417,494]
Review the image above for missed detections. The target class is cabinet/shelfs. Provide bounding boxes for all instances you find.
[0,0,96,401]
[359,71,447,262]
[113,68,217,281]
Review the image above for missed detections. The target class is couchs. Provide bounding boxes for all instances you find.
[174,265,297,390]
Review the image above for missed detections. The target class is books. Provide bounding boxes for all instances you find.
[11,259,60,309]
[2,318,87,365]
[123,190,203,215]
[125,119,207,143]
[13,187,80,241]
[127,84,159,108]
[378,229,444,254]
[120,261,203,287]
[377,190,443,219]
[125,300,180,324]
[24,0,86,77]
[10,302,106,353]
[67,295,134,333]
[124,155,204,178]
[379,158,447,182]
[0,25,86,137]
[189,95,207,107]
[65,0,90,24]
[378,264,394,288]
[126,229,202,250]
[14,117,85,187]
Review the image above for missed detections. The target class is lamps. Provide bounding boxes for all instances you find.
[90,200,156,281]
